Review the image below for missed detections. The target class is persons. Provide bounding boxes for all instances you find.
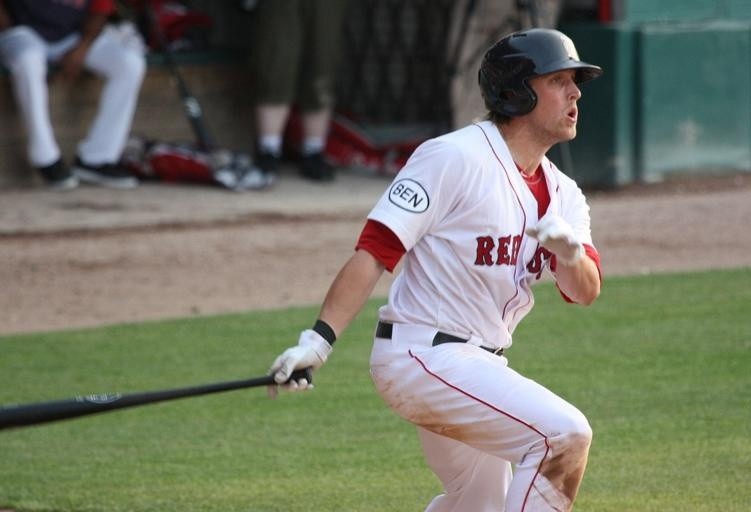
[249,0,350,182]
[262,27,604,512]
[0,0,149,194]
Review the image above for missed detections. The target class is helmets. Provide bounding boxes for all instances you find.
[478,27,604,118]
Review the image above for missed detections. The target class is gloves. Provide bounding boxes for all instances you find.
[266,328,334,401]
[527,216,584,266]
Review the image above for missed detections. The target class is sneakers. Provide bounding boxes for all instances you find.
[292,147,337,181]
[37,159,77,192]
[71,156,140,189]
[258,150,284,176]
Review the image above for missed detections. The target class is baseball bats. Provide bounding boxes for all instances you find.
[0,369,312,430]
[131,0,216,152]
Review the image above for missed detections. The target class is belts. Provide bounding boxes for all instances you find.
[374,320,505,358]
